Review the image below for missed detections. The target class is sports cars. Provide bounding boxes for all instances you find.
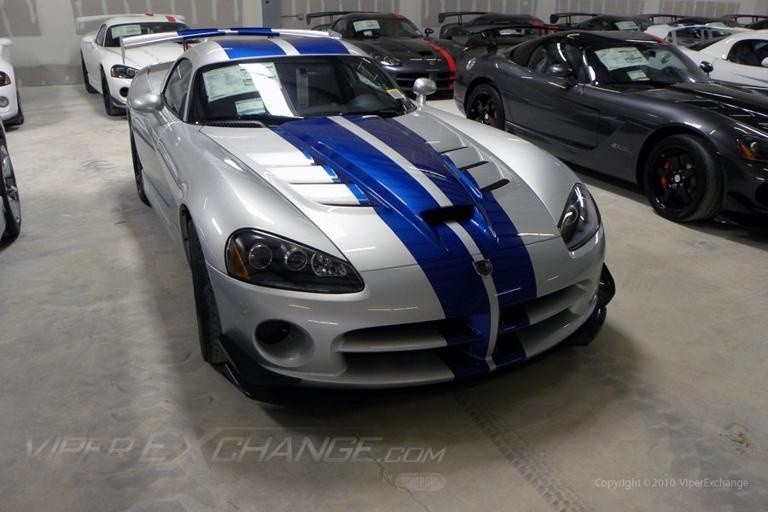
[0,6,768,410]
